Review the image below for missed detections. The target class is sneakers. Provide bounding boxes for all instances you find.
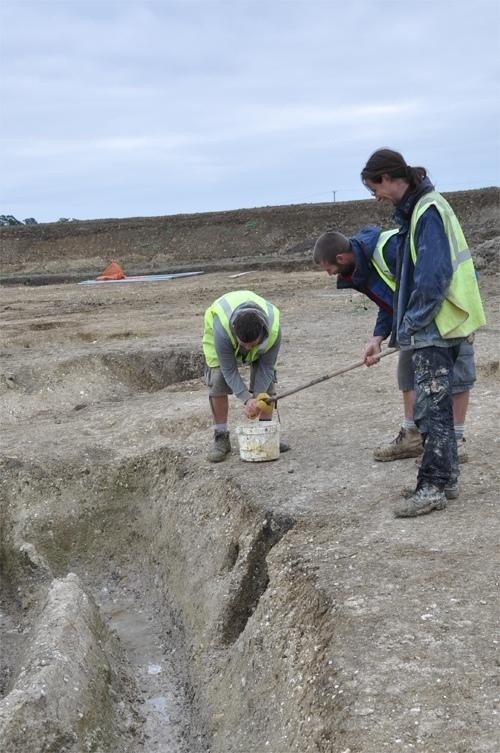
[393,480,448,517]
[455,435,469,466]
[401,480,460,499]
[373,425,425,462]
[207,428,231,462]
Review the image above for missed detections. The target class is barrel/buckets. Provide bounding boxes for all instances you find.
[236,421,280,462]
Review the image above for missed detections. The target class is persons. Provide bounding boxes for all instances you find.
[361,148,485,518]
[203,286,289,463]
[313,227,476,466]
